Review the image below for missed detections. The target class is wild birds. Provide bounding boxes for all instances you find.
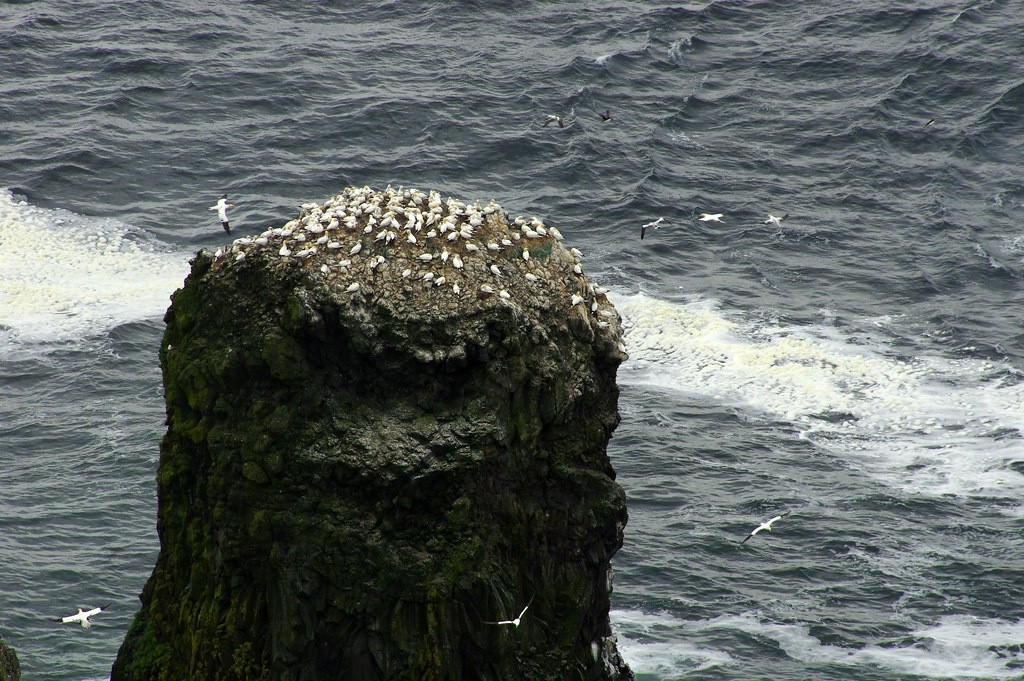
[208,193,233,236]
[542,114,563,129]
[214,184,616,329]
[761,214,789,227]
[589,109,612,124]
[479,591,536,628]
[640,217,672,240]
[740,510,792,543]
[699,213,726,224]
[44,601,111,630]
